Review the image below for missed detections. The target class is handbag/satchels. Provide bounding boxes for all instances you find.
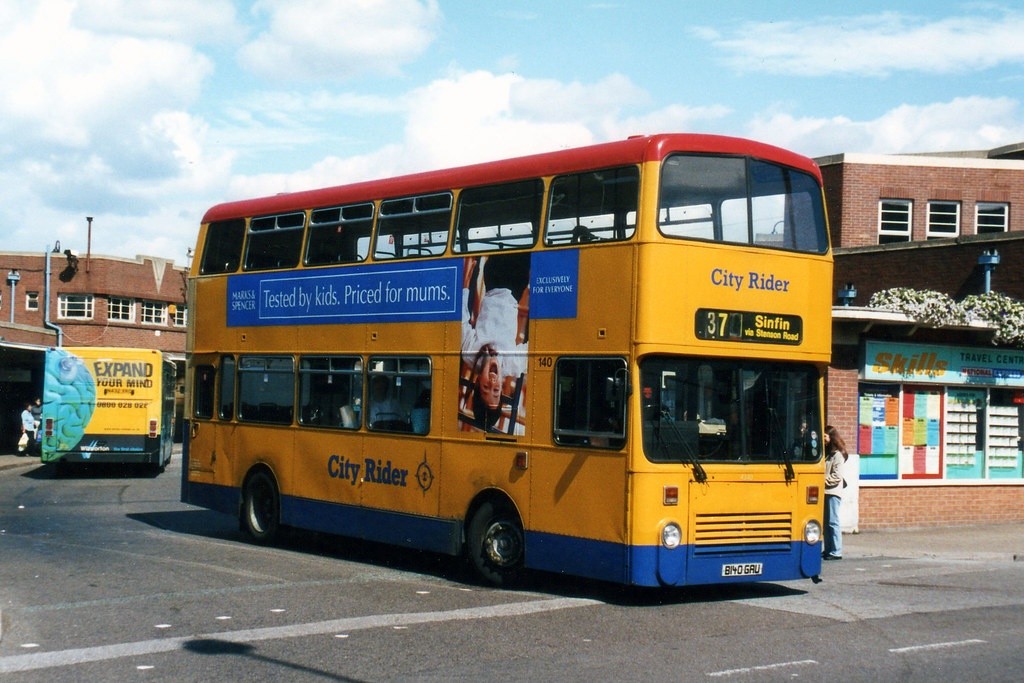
[18,433,29,451]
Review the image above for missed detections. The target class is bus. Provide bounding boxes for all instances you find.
[40,347,184,476]
[178,134,835,591]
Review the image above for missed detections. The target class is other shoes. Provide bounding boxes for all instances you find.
[824,555,842,560]
[821,550,829,557]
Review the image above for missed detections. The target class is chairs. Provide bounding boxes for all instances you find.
[223,225,613,272]
[202,402,412,435]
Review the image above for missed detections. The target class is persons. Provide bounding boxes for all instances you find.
[360,376,408,433]
[20,404,38,457]
[571,227,598,244]
[30,399,43,436]
[461,254,528,427]
[822,425,848,561]
